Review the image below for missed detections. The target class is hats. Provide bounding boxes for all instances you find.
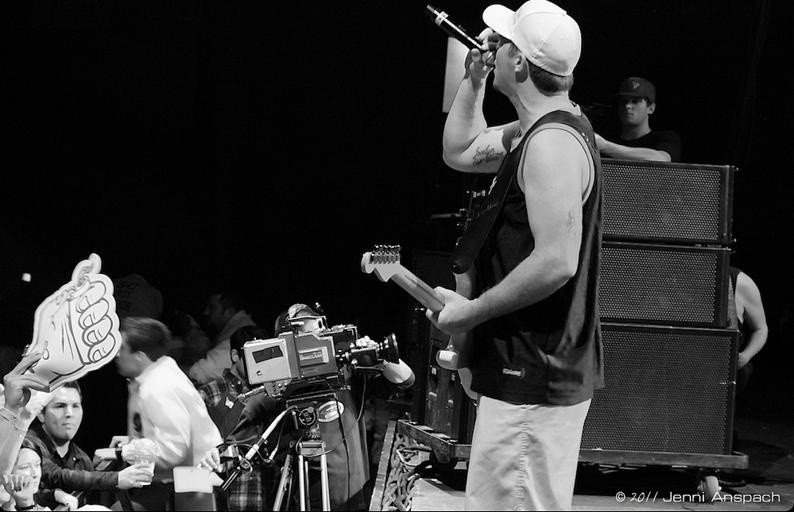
[618,77,655,103]
[484,0,582,77]
[281,304,317,329]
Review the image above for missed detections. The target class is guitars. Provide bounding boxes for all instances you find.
[361,245,479,402]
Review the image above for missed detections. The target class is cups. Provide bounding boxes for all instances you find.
[135,454,157,486]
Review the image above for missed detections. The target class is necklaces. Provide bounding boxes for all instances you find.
[484,117,523,196]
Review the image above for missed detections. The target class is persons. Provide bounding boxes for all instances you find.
[592,75,681,164]
[196,301,416,512]
[420,0,611,512]
[692,241,768,487]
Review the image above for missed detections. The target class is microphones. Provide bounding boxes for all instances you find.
[421,2,496,71]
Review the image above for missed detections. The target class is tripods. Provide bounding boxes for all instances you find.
[273,435,333,511]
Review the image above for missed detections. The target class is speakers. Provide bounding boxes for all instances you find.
[597,157,738,242]
[594,239,733,331]
[572,324,739,456]
